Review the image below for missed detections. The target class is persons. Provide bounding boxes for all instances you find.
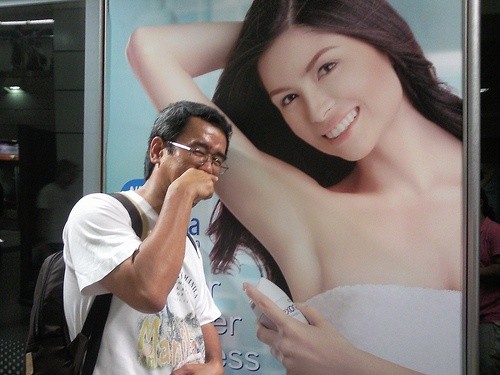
[126,0,466,375]
[480,188,500,375]
[61,100,231,374]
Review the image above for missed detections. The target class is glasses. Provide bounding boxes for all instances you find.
[165,139,229,172]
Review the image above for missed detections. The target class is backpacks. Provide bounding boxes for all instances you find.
[24,192,199,374]
